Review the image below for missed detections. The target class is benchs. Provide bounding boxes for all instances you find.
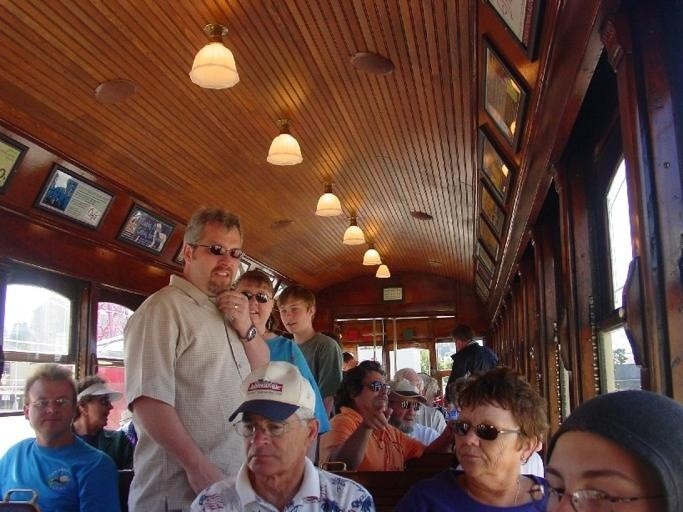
[403,453,460,469]
[323,460,466,512]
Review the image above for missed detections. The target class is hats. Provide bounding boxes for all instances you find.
[228,361,316,421]
[388,378,429,403]
[78,384,123,402]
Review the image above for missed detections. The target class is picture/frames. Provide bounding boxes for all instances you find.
[272,279,290,302]
[34,163,115,231]
[172,240,185,267]
[0,131,29,193]
[118,199,175,261]
[232,259,251,287]
[471,0,545,302]
[254,268,274,283]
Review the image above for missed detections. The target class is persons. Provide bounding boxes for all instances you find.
[319,358,453,469]
[277,285,342,420]
[234,269,332,434]
[343,352,357,370]
[444,324,502,406]
[528,390,682,512]
[395,366,550,512]
[71,376,137,470]
[0,362,122,511]
[190,361,374,511]
[123,207,270,510]
[387,368,447,446]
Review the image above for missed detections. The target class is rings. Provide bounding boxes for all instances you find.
[230,316,234,321]
[234,304,237,311]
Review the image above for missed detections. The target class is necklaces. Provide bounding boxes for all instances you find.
[465,475,520,505]
[262,331,268,336]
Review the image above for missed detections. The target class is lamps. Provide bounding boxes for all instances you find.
[259,116,303,166]
[315,179,342,217]
[374,260,390,278]
[342,212,365,246]
[186,23,240,89]
[362,241,382,266]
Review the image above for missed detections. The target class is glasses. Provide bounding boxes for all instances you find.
[365,381,387,393]
[233,421,294,438]
[528,481,664,511]
[450,419,518,441]
[244,290,271,299]
[196,243,245,259]
[394,400,421,411]
[95,398,112,406]
[31,398,71,411]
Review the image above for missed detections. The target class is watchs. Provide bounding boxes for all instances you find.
[239,321,257,342]
